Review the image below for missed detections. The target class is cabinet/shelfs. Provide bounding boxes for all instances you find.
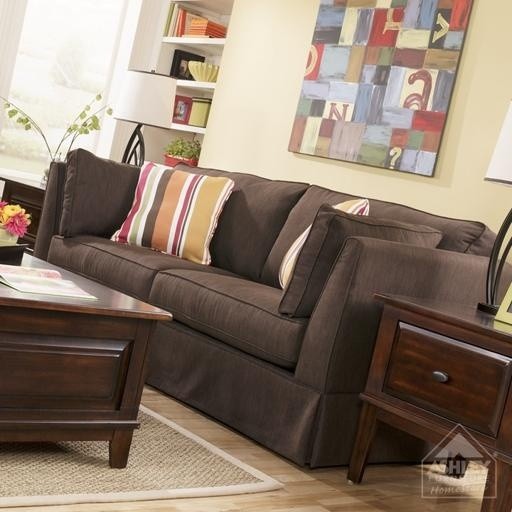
[95,0,235,166]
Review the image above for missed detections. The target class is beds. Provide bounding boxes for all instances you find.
[32,149,512,467]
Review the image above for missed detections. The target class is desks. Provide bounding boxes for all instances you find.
[1,175,45,251]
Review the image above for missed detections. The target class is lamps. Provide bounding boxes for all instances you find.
[111,68,175,166]
[475,105,512,315]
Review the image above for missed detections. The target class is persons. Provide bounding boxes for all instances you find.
[180,61,188,77]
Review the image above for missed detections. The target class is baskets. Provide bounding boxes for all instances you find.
[188,61,219,82]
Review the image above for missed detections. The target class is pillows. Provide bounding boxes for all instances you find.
[57,148,496,316]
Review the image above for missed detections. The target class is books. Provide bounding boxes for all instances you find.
[0,262,100,300]
[163,3,227,40]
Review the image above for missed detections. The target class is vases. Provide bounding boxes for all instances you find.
[0,229,20,246]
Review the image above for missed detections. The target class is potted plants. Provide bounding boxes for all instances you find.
[162,133,205,167]
[1,80,112,186]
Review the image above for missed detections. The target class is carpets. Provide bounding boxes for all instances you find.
[0,400,286,511]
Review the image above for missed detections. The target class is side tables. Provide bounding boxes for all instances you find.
[346,292,512,511]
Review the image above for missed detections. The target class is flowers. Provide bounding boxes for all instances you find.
[1,200,32,237]
